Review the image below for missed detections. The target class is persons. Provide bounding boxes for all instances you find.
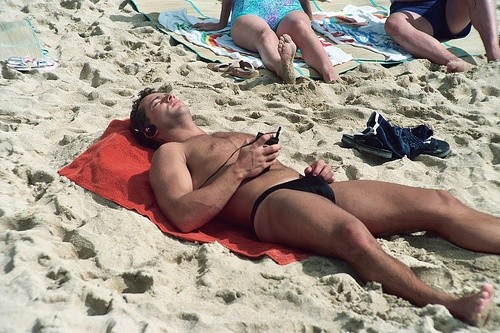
[384,0,500,73]
[129,86,499,327]
[193,0,339,84]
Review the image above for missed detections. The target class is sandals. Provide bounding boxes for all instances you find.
[207,60,260,78]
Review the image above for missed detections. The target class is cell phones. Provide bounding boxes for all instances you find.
[255,132,279,171]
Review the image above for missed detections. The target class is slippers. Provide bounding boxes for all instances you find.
[417,138,452,156]
[4,58,54,71]
[342,127,392,158]
